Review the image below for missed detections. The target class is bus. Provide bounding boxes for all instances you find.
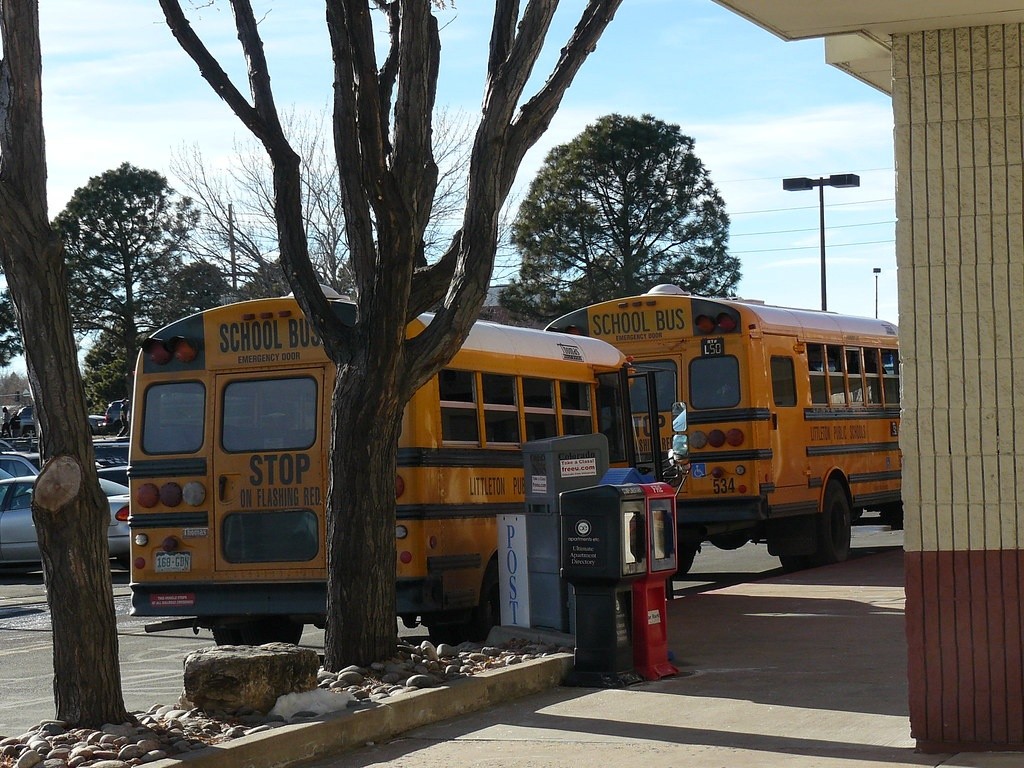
[542,283,900,580]
[126,284,690,646]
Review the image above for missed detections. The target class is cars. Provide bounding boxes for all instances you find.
[0,475,130,570]
[0,399,130,488]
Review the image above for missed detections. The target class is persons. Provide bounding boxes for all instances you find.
[1,407,11,438]
[116,397,129,436]
[8,410,21,437]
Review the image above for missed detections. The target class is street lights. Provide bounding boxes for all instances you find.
[782,173,860,311]
[873,267,881,318]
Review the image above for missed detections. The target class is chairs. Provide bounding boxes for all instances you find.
[12,492,32,510]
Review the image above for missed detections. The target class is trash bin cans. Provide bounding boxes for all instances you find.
[521,430,611,635]
